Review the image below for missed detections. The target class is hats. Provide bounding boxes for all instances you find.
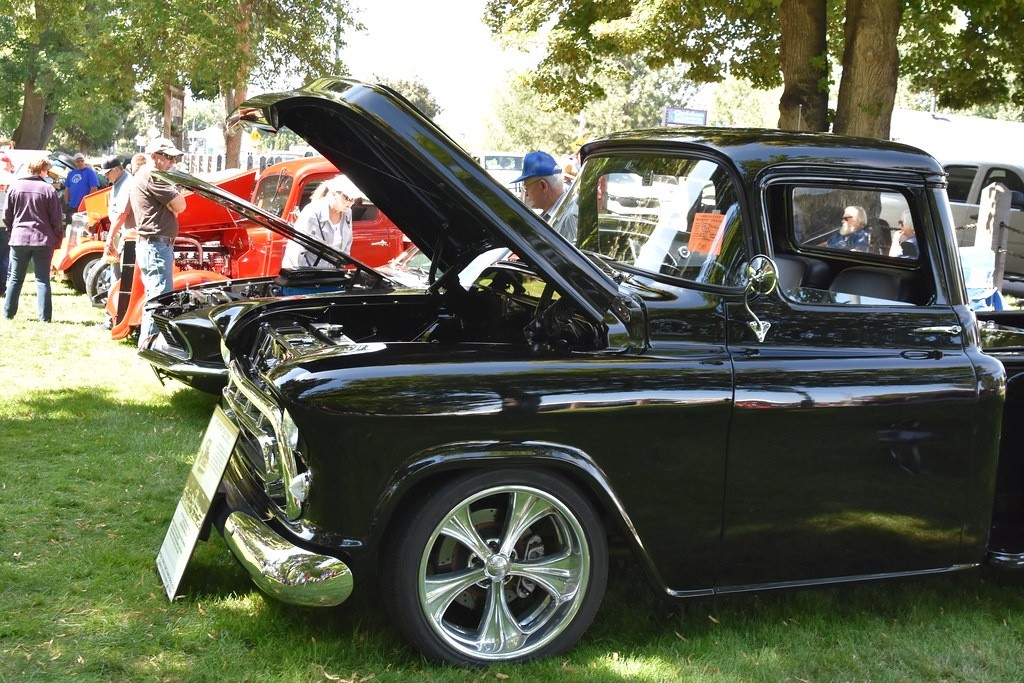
[145,138,184,157]
[98,155,120,175]
[73,153,84,161]
[331,174,369,200]
[511,150,562,184]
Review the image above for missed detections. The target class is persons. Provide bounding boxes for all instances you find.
[3,158,64,323]
[132,153,146,176]
[818,205,870,253]
[898,208,920,260]
[0,158,16,299]
[98,155,137,331]
[63,152,101,240]
[510,150,579,248]
[282,175,362,271]
[130,137,187,354]
[10,141,16,150]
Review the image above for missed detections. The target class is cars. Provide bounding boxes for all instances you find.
[0,75,1024,671]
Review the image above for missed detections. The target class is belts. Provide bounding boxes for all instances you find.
[139,236,175,246]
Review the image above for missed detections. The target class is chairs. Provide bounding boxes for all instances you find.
[739,254,828,293]
[823,266,927,306]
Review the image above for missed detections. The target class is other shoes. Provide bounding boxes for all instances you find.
[0,292,7,298]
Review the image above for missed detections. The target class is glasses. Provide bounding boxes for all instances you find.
[898,221,904,226]
[521,179,548,192]
[155,152,174,160]
[336,190,354,202]
[842,217,852,221]
